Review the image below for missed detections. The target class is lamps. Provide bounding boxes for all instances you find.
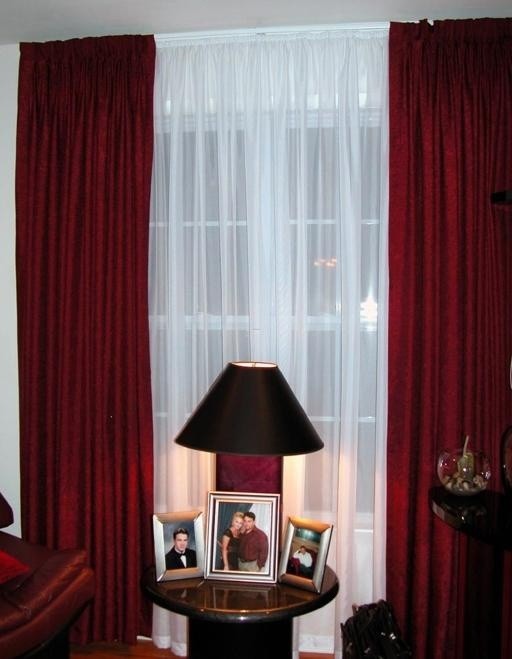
[176,362,323,494]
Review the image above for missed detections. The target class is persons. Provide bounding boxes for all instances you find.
[219,511,245,571]
[238,511,268,572]
[293,546,314,579]
[164,527,198,571]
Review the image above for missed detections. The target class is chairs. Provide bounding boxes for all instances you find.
[0,493,94,659]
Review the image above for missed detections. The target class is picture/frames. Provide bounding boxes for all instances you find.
[150,491,333,595]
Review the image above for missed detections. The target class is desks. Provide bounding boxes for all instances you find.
[429,484,512,659]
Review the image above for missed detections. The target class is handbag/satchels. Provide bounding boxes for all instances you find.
[342,599,406,658]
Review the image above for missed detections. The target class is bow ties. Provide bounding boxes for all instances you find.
[178,553,186,558]
